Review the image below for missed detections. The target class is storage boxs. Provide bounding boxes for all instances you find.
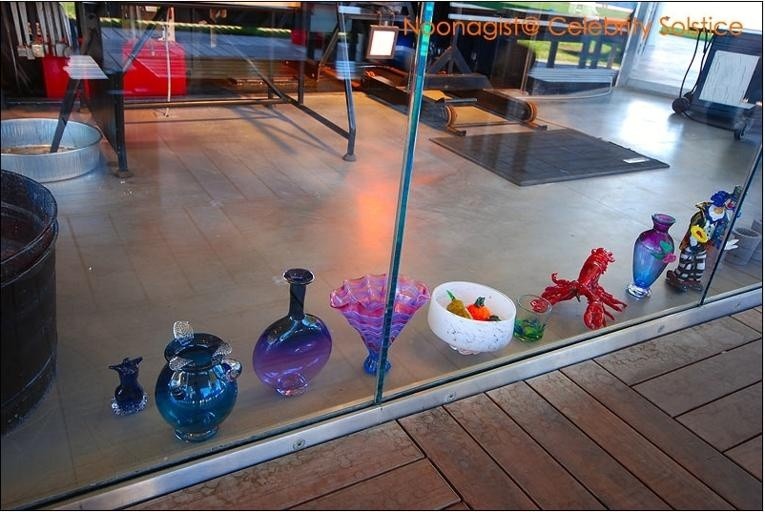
[122,37,186,98]
[42,54,97,98]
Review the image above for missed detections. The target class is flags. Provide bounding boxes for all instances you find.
[488,313,502,321]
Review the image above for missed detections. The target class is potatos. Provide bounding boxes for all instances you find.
[447,300,473,319]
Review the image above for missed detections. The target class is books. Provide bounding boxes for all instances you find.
[709,185,742,249]
[531,246,629,332]
[666,188,742,296]
[443,290,471,320]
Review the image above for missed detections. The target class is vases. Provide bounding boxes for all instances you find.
[108,211,678,444]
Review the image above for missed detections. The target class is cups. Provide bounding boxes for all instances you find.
[515,295,552,343]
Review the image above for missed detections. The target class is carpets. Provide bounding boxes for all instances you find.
[428,128,670,186]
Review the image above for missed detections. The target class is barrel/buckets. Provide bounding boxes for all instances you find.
[0,168,59,439]
